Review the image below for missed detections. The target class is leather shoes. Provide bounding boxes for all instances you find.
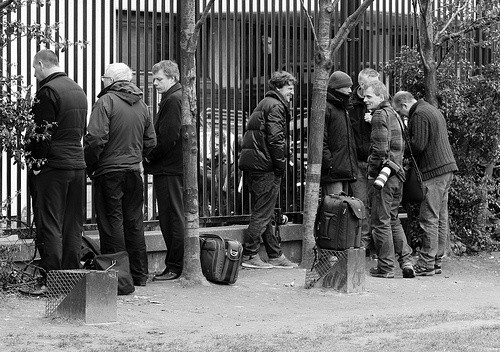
[153,271,179,280]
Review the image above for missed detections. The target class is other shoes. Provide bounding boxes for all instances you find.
[369,266,395,278]
[402,267,415,278]
[134,279,147,286]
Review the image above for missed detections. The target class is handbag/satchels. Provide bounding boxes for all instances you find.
[199,233,243,284]
[400,167,428,205]
[93,250,135,295]
[314,191,366,250]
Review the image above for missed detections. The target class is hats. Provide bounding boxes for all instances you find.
[328,70,354,89]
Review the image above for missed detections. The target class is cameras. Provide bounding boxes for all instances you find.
[374,160,401,190]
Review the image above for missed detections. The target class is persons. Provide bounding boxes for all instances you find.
[362,83,416,278]
[84,62,157,295]
[320,70,359,196]
[237,71,298,268]
[143,60,183,282]
[20,49,87,295]
[394,92,458,277]
[198,133,242,216]
[349,69,380,259]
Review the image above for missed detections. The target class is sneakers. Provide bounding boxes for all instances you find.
[413,263,435,276]
[268,253,299,268]
[242,253,274,269]
[434,262,442,274]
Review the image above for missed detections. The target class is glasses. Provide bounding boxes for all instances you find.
[100,75,114,83]
